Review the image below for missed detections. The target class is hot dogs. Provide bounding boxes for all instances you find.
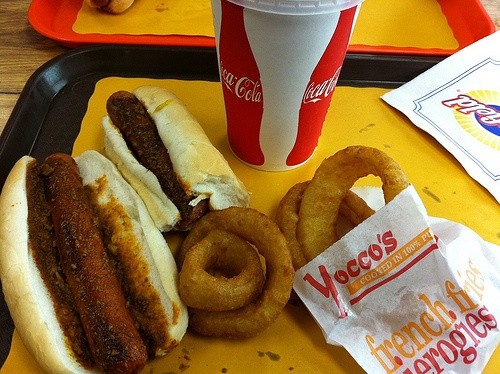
[103,84,250,235]
[85,0,136,13]
[0,149,191,374]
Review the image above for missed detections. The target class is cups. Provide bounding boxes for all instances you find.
[208,0,364,173]
